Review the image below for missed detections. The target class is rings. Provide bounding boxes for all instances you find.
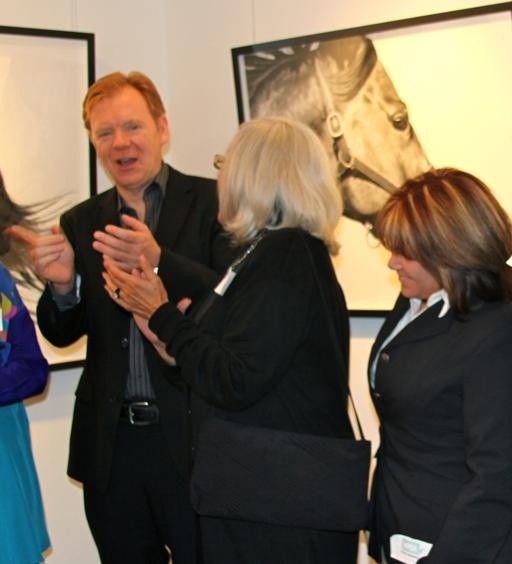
[112,288,121,299]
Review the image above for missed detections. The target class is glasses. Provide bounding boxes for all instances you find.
[213,153,224,170]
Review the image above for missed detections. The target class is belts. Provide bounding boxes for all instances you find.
[120,400,161,425]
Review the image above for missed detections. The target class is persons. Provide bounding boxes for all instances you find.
[364,167,512,564]
[101,115,359,564]
[11,69,254,564]
[0,263,52,564]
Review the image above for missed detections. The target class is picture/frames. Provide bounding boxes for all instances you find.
[229,2,511,321]
[0,24,100,370]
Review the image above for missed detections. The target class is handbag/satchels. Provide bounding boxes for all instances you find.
[189,228,373,533]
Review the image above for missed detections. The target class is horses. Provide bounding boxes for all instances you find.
[244,35,435,240]
